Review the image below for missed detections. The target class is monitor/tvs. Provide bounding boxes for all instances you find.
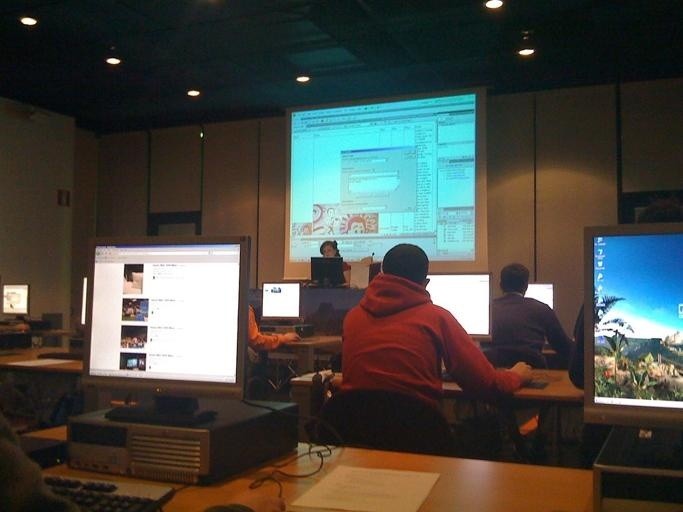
[425,270,492,342]
[82,235,252,401]
[524,282,557,313]
[77,272,88,327]
[1,283,31,315]
[311,256,346,285]
[583,221,683,431]
[260,282,302,319]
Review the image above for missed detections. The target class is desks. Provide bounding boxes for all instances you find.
[280,335,343,437]
[0,346,84,421]
[539,348,558,356]
[290,367,586,468]
[13,426,602,512]
[259,351,333,363]
[0,329,78,346]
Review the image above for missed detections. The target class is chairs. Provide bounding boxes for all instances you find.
[483,345,548,372]
[308,388,457,459]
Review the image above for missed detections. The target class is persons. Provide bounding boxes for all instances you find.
[245,305,302,382]
[341,242,533,413]
[489,263,575,370]
[320,240,351,288]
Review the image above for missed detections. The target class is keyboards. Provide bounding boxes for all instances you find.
[43,470,176,512]
